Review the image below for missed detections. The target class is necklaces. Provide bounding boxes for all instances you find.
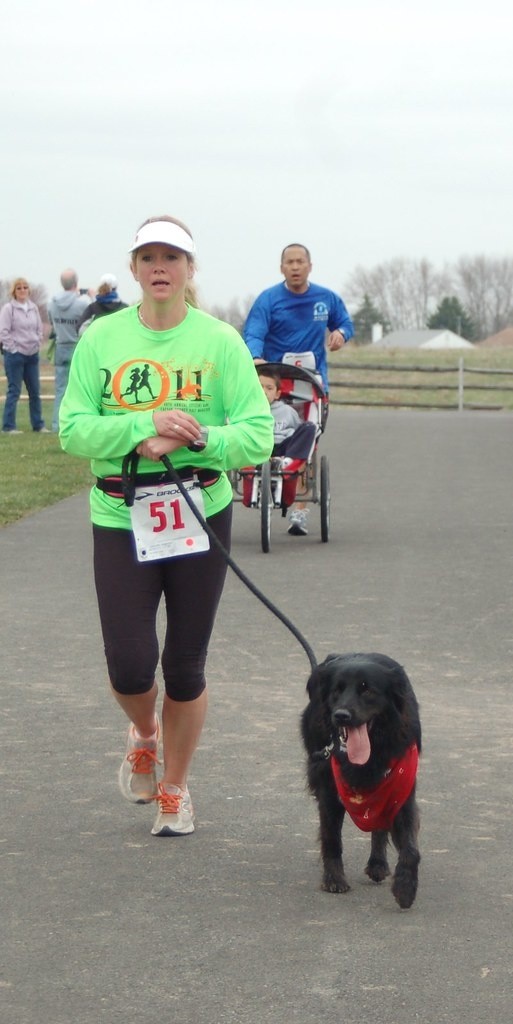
[138,302,156,331]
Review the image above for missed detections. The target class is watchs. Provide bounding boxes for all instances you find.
[337,328,346,342]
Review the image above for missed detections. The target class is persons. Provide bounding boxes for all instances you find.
[254,368,316,473]
[57,216,275,835]
[48,268,98,430]
[0,278,50,433]
[242,244,354,536]
[74,281,130,330]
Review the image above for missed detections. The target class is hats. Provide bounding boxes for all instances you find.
[127,219,195,255]
[99,273,117,289]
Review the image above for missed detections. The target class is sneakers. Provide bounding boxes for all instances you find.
[150,774,195,837]
[286,508,308,536]
[118,712,160,804]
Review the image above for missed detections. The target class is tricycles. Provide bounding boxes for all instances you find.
[231,364,333,555]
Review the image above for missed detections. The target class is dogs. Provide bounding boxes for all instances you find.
[297,652,427,911]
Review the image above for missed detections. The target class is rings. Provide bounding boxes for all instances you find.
[174,425,179,431]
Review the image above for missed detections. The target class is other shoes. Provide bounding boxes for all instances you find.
[0,430,22,434]
[271,458,283,471]
[36,427,51,433]
